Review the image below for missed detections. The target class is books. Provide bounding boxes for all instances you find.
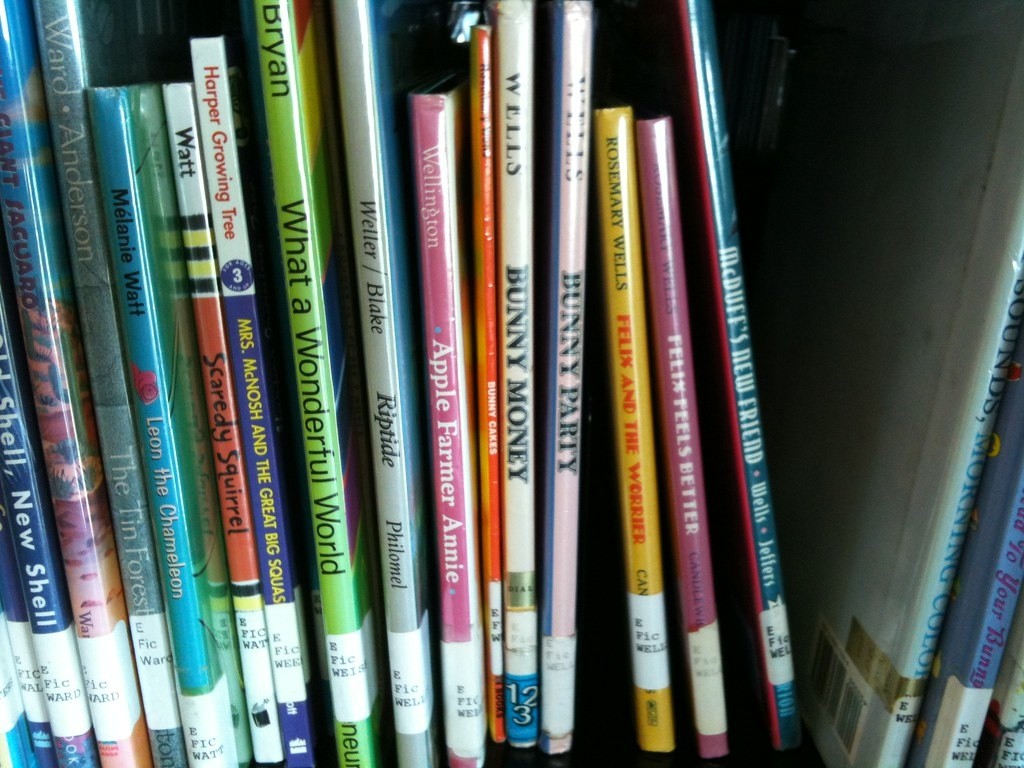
[0,0,1024,768]
